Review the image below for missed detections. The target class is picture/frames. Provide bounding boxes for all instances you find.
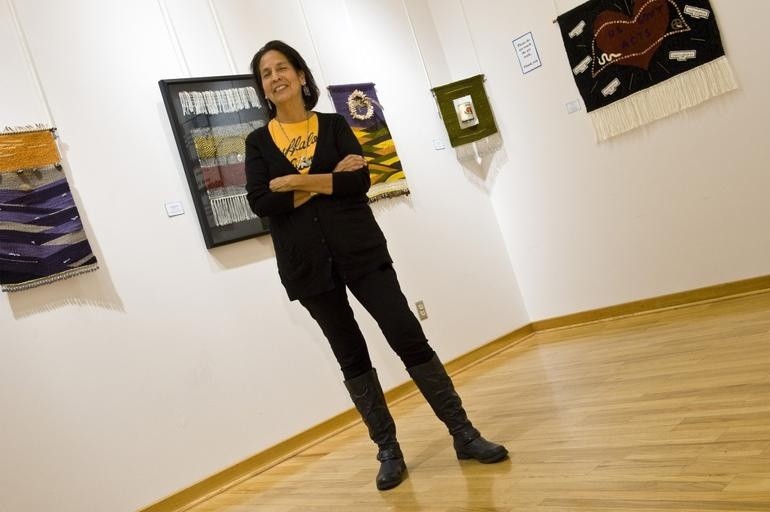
[158,74,273,249]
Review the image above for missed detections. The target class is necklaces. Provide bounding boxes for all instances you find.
[274,110,311,163]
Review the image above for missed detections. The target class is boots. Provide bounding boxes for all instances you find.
[343,367,406,490]
[405,350,507,464]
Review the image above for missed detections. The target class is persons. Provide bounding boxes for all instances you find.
[241,37,512,492]
